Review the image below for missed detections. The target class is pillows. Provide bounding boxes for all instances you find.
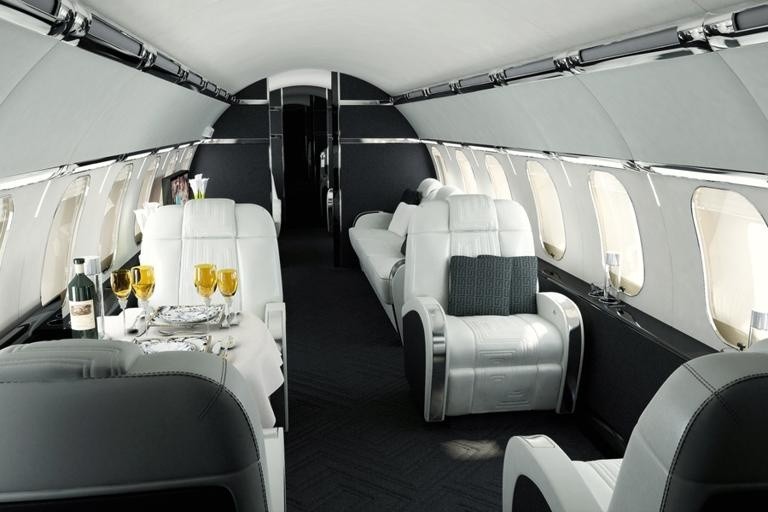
[475,253,540,317]
[388,187,423,236]
[446,254,513,317]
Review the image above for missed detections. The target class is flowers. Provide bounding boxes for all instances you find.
[188,172,209,200]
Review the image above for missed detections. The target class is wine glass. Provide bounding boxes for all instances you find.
[193,263,219,340]
[216,268,237,334]
[130,265,156,327]
[110,269,133,339]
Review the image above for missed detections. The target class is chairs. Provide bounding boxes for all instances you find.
[400,193,584,427]
[137,198,291,436]
[1,338,288,512]
[499,338,768,511]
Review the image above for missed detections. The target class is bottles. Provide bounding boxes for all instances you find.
[748,307,767,347]
[67,258,99,341]
[602,251,622,302]
[70,257,107,339]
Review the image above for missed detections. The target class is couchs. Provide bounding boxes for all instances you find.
[347,177,457,335]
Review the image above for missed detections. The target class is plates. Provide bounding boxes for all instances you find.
[131,336,207,356]
[155,303,221,326]
